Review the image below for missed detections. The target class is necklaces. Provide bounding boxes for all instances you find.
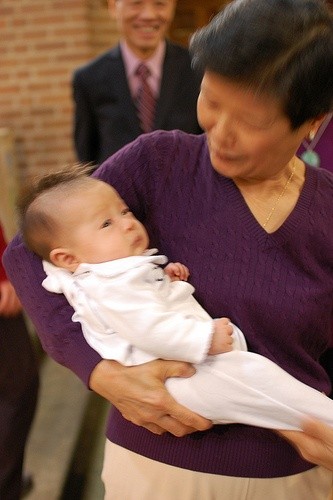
[265,158,298,227]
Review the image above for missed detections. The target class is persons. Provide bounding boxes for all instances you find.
[0,0,333,500]
[16,162,333,431]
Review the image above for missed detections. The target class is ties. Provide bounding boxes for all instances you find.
[133,64,158,134]
